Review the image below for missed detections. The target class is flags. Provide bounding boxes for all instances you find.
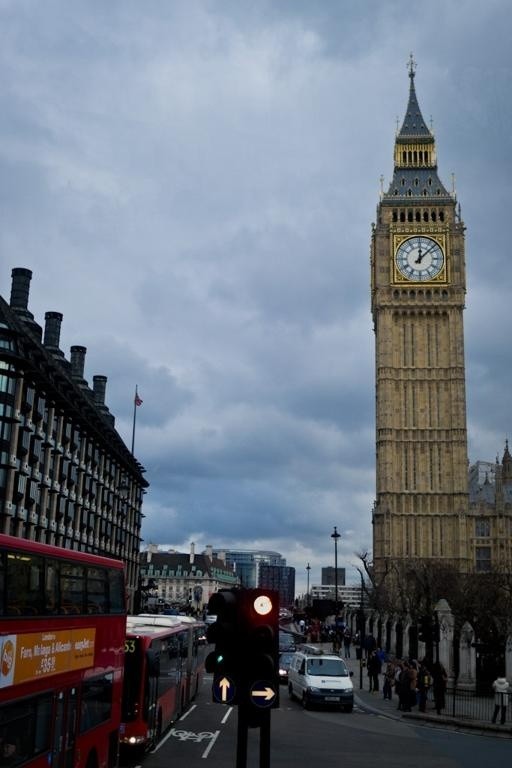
[135,392,144,407]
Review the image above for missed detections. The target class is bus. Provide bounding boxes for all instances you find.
[120,613,205,754]
[0,534,128,768]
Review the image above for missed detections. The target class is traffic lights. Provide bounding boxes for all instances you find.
[202,588,240,707]
[241,588,281,710]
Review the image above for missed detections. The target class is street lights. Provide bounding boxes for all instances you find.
[306,563,311,604]
[330,526,340,602]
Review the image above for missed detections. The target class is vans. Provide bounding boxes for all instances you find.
[288,644,354,713]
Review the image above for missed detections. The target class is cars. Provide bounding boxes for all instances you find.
[278,632,297,682]
[279,608,288,617]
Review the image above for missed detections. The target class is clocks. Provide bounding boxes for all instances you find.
[390,233,450,289]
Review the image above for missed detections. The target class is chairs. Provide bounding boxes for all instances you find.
[0,594,123,613]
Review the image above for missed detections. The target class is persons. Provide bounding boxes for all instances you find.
[0,737,17,765]
[319,623,447,716]
[488,669,510,726]
